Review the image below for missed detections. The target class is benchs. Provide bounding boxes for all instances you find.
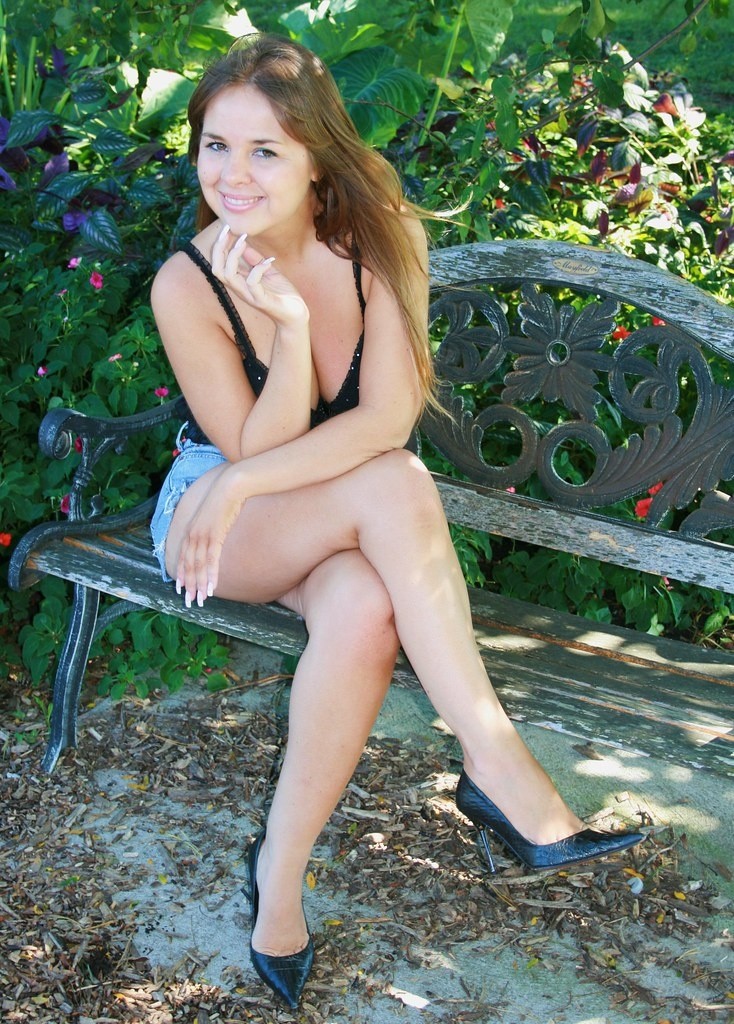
[8,239,734,782]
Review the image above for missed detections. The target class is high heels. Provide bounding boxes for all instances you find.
[248,832,315,1010]
[455,766,645,873]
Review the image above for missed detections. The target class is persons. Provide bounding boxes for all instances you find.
[149,36,643,1010]
